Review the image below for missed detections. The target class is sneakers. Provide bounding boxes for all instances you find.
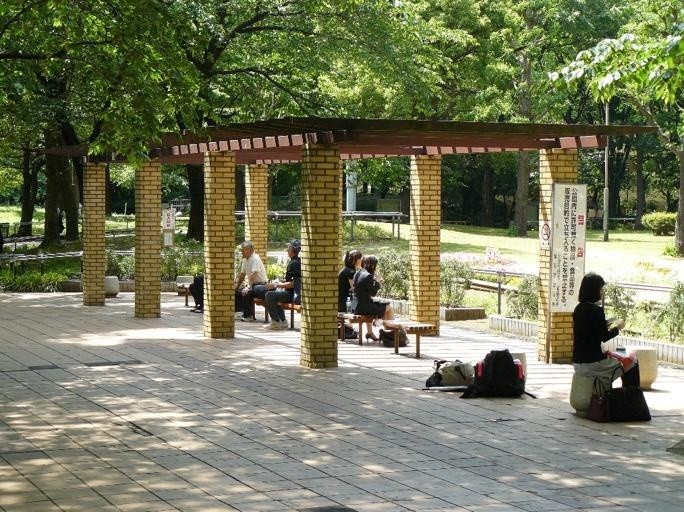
[263,320,288,329]
[235,314,253,322]
[191,303,202,314]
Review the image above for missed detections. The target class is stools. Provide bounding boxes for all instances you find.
[569,370,613,419]
[629,345,660,391]
[507,350,527,385]
[104,276,119,298]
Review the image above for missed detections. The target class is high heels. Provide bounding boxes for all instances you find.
[366,333,379,342]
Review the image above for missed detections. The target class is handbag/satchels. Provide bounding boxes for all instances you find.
[379,328,410,347]
[588,366,651,423]
[426,350,525,399]
[338,319,359,339]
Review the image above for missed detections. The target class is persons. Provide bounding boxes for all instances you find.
[186,274,203,312]
[570,272,638,396]
[338,249,362,311]
[262,239,300,329]
[234,241,267,324]
[351,255,396,342]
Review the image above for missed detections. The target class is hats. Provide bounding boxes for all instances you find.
[287,239,301,251]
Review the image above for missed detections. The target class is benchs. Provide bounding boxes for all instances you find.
[177,285,301,328]
[382,317,440,356]
[2,220,46,251]
[332,312,380,348]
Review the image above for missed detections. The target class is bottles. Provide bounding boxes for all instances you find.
[345,296,352,315]
